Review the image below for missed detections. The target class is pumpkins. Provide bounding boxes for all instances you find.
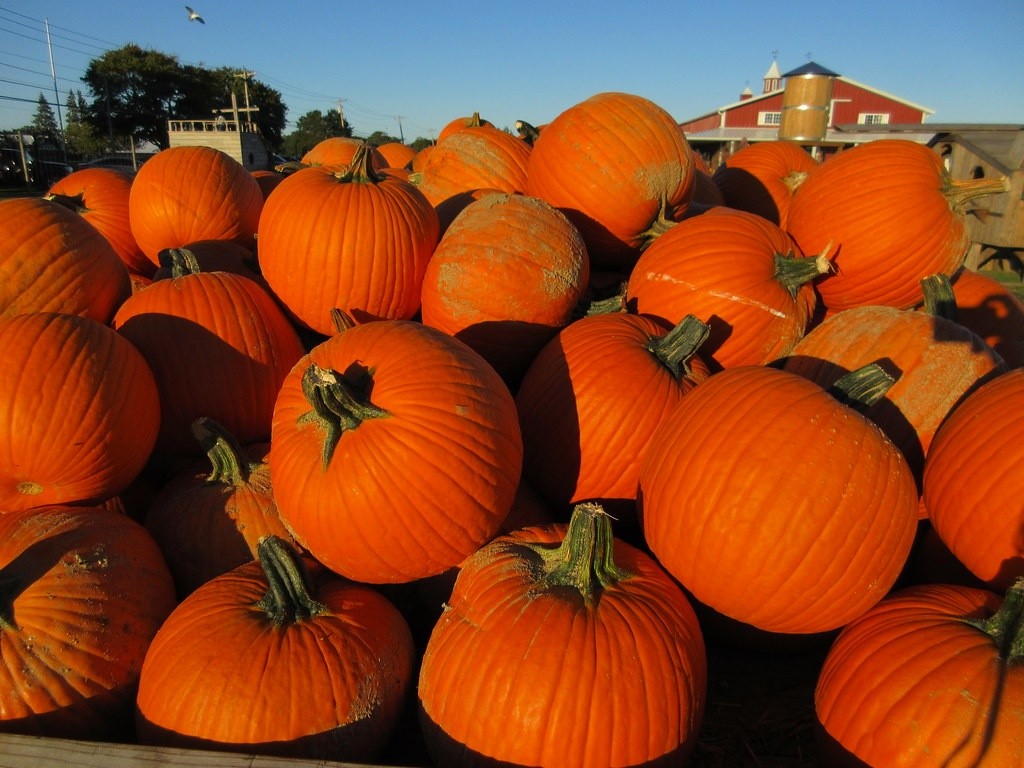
[0,91,1024,768]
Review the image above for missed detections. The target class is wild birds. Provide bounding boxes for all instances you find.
[184,6,206,26]
